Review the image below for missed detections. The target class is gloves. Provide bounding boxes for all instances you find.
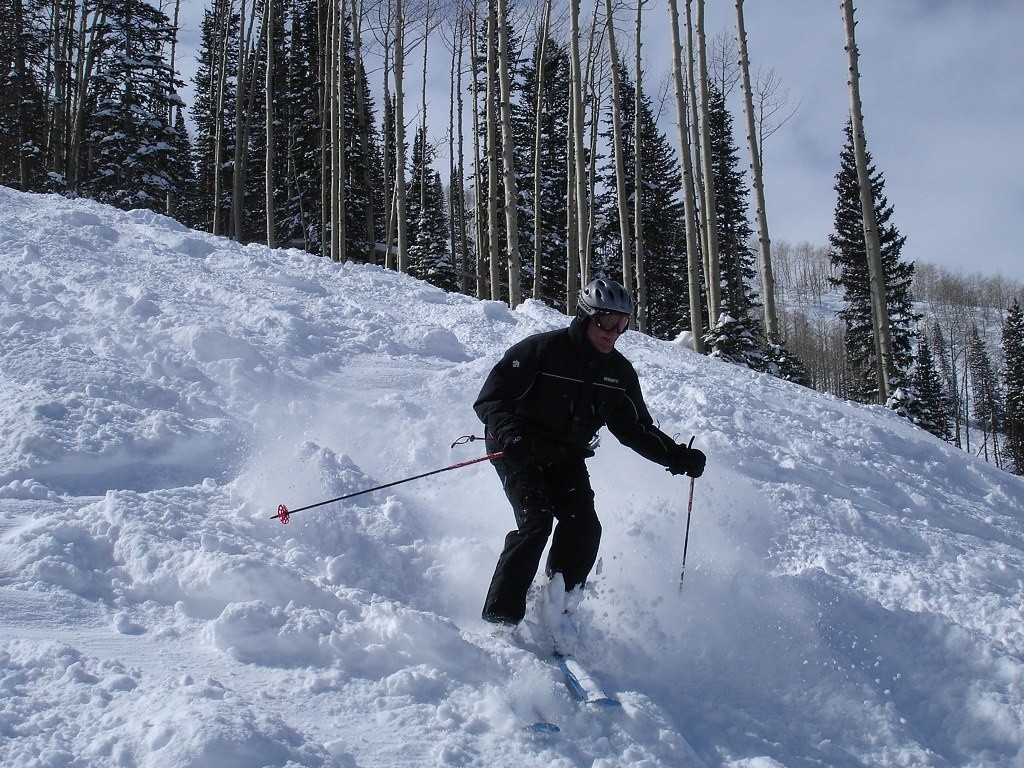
[497,425,528,455]
[669,445,707,479]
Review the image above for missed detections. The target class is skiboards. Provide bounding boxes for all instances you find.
[501,639,624,738]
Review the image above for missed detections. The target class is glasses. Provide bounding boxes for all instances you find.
[591,312,630,334]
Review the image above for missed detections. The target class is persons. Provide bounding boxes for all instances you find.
[473,279,706,634]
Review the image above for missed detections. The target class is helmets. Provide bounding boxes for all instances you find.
[577,279,634,320]
